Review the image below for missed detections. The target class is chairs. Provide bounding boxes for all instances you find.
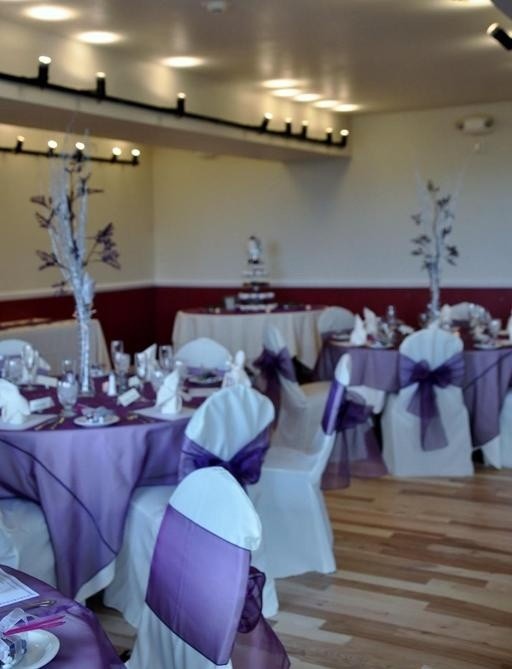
[124,467,289,669]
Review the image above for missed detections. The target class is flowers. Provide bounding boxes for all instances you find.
[30,106,119,391]
[410,177,459,328]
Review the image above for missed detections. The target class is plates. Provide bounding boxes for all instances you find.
[72,415,122,428]
[0,626,61,669]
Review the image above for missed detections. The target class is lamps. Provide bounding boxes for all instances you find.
[486,22,512,50]
[0,56,349,148]
[0,135,141,166]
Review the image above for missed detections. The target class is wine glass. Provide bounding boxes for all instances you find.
[0,340,174,417]
[488,319,501,348]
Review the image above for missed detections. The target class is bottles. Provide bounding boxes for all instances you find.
[387,306,397,330]
